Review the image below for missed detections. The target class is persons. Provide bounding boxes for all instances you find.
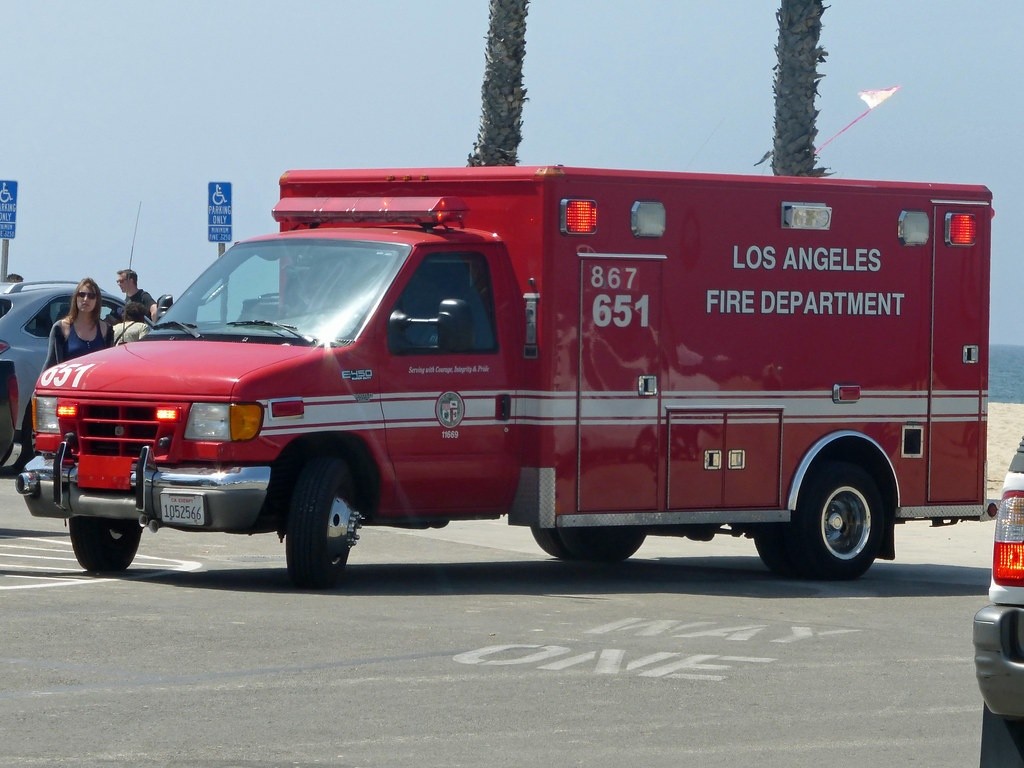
[6,273,24,283]
[115,269,159,325]
[43,276,114,369]
[113,299,152,348]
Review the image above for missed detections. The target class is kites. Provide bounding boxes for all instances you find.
[856,86,900,108]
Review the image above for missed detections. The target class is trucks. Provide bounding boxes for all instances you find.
[14,166,995,591]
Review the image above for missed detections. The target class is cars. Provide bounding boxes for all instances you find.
[972,433,1024,719]
[0,279,155,476]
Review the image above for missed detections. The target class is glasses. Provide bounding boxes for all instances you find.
[78,291,97,299]
[116,279,128,283]
